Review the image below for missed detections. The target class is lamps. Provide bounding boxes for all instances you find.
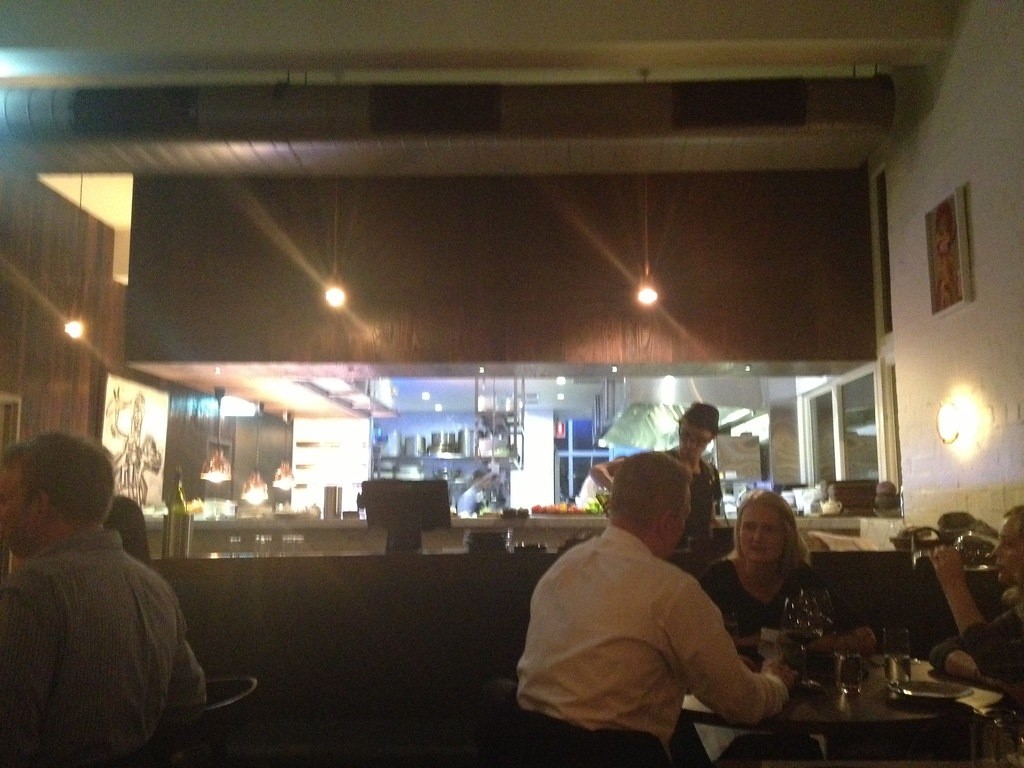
[198,386,298,503]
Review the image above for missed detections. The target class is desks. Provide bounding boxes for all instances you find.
[679,651,1005,768]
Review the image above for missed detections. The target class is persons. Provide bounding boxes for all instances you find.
[456,469,488,514]
[696,489,878,654]
[928,503,1024,707]
[516,450,800,768]
[577,459,624,506]
[0,434,207,768]
[591,402,722,572]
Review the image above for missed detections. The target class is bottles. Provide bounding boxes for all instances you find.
[167,464,188,515]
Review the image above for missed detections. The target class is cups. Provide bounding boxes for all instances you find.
[323,486,343,520]
[882,627,911,689]
[971,707,1024,768]
[838,654,863,695]
[162,514,194,560]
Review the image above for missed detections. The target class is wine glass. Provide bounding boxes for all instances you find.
[784,584,837,696]
[910,531,1000,573]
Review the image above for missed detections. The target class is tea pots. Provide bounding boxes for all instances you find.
[820,498,842,513]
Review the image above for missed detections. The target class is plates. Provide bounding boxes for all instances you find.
[887,680,974,704]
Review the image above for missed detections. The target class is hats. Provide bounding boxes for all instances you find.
[684,404,719,434]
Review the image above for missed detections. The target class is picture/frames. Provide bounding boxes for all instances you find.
[924,189,971,317]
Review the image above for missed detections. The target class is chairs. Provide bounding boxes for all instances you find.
[152,673,257,768]
